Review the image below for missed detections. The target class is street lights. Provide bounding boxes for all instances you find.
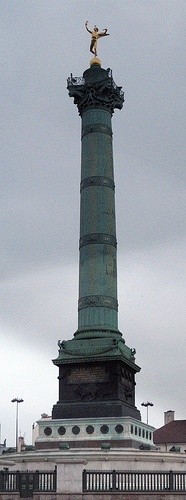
[141,401,154,425]
[12,397,23,453]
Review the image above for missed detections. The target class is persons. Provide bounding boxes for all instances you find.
[85,21,110,57]
